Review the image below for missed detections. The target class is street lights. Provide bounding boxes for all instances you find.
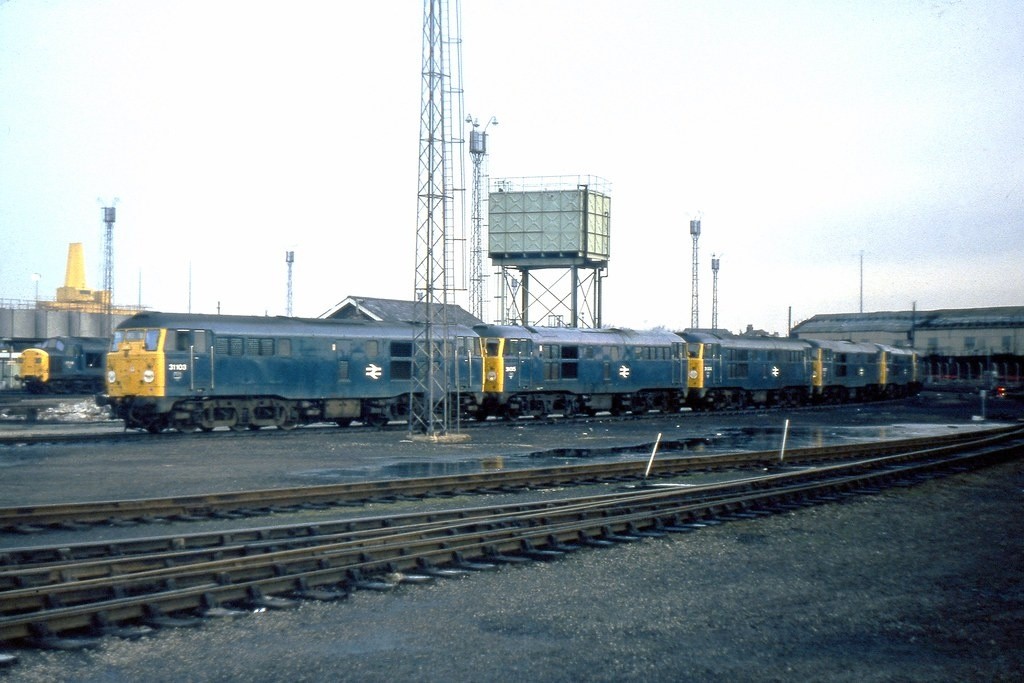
[465,114,499,321]
[99,205,118,341]
[689,218,700,331]
[710,252,722,330]
[285,250,296,318]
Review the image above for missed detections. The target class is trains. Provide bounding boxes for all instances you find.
[95,309,924,434]
[14,334,113,396]
